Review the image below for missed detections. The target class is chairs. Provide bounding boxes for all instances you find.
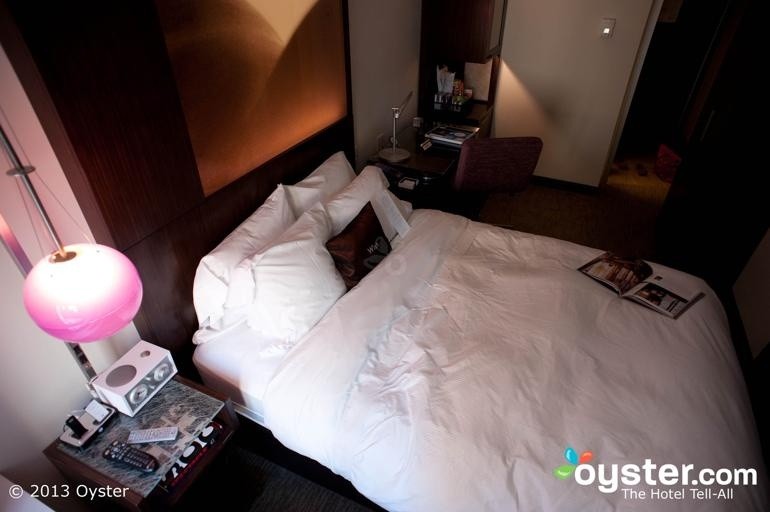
[443,136,545,203]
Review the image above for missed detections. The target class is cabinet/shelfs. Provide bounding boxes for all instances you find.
[421,0,510,64]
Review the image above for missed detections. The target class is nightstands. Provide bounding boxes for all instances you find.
[41,376,263,511]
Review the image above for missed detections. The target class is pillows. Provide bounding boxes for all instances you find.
[189,149,413,356]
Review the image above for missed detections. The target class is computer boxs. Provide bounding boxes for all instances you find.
[91,339,180,418]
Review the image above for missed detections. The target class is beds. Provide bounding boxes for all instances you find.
[120,115,764,511]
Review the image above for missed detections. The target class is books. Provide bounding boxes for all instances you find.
[424,119,480,146]
[576,249,707,321]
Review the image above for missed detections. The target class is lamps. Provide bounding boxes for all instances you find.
[0,120,145,347]
[376,89,412,164]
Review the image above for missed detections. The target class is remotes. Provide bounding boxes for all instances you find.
[126,426,179,445]
[103,439,159,474]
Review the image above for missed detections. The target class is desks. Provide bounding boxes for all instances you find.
[373,96,494,176]
